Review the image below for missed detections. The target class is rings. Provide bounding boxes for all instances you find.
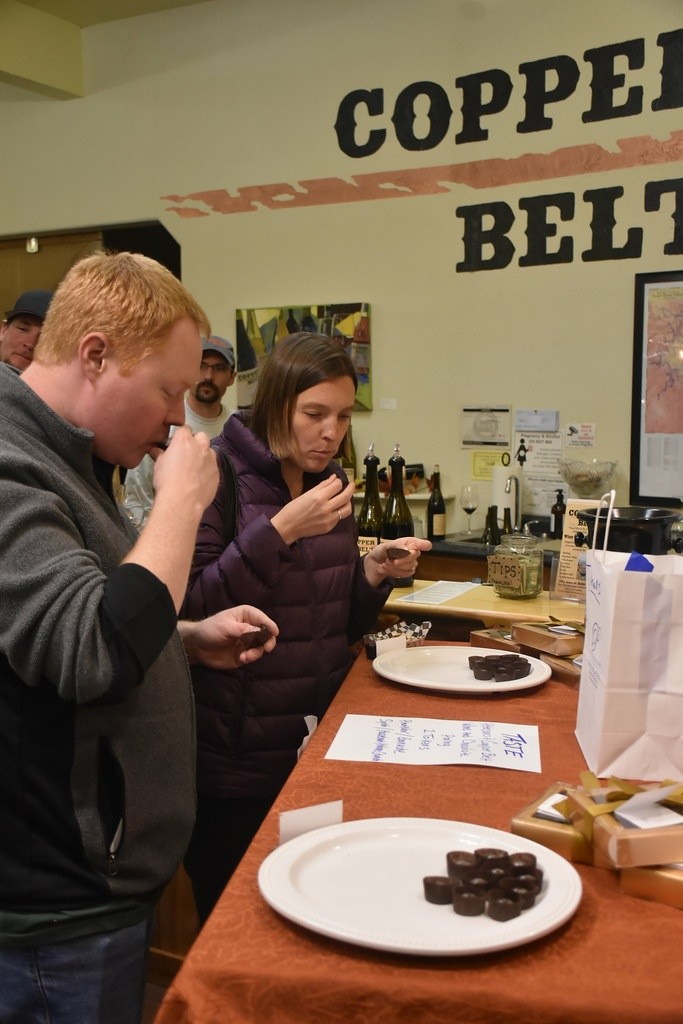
[409,565,415,572]
[337,509,341,521]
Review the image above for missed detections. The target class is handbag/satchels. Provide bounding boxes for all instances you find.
[574,488,683,783]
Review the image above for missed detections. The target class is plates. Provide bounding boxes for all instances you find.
[372,646,552,695]
[258,818,583,957]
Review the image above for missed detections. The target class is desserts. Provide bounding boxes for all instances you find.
[468,654,531,682]
[423,848,543,922]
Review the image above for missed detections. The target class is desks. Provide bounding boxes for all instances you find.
[384,575,586,640]
[149,637,683,1024]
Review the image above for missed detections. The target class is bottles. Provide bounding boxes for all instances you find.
[357,444,384,558]
[427,465,446,542]
[482,505,513,546]
[286,306,332,337]
[494,534,543,597]
[350,303,370,374]
[384,444,414,588]
[236,309,266,409]
[332,416,356,483]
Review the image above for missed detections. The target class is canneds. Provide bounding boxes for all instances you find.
[494,534,543,599]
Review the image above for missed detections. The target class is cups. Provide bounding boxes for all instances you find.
[115,484,145,531]
[413,517,423,539]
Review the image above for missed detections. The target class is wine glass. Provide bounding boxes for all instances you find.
[461,487,479,535]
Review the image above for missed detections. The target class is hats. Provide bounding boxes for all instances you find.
[200,336,235,366]
[4,290,53,321]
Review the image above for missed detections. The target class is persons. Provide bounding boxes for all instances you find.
[178,331,432,927]
[0,252,279,1024]
[0,290,54,371]
[122,334,237,533]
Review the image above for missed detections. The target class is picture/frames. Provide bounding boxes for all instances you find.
[628,268,683,507]
